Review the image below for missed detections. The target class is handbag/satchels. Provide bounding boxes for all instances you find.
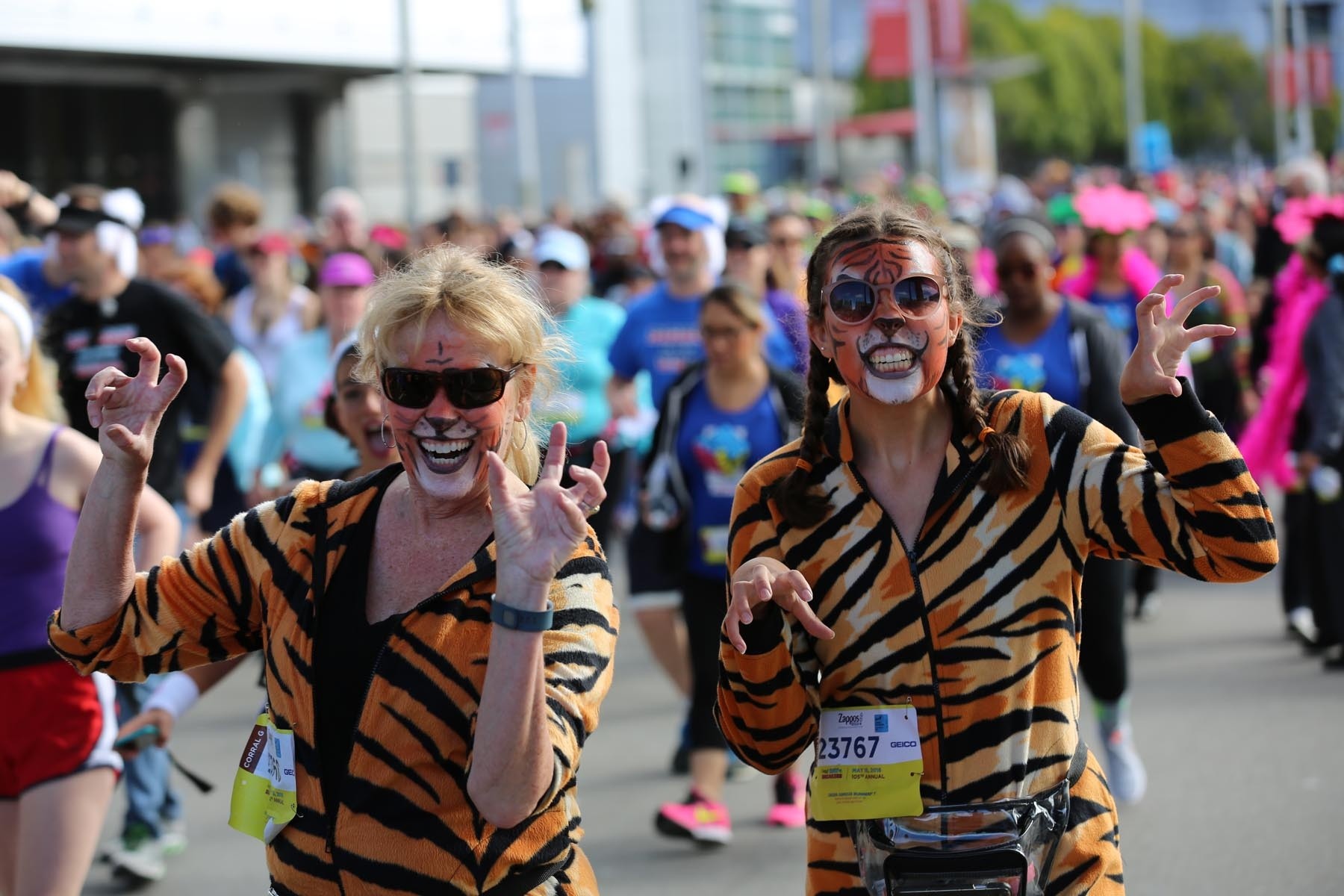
[846,748,1088,896]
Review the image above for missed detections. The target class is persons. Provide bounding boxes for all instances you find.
[0,153,1344,896]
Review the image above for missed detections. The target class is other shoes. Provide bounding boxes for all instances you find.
[90,822,184,890]
[1097,720,1147,805]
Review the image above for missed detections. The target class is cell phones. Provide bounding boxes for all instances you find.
[113,723,162,756]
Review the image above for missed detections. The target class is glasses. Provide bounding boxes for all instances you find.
[381,362,528,409]
[700,322,753,340]
[819,273,953,325]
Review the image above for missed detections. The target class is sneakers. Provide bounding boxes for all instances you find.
[656,786,732,846]
[766,769,808,828]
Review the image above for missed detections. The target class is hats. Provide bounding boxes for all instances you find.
[530,225,591,271]
[36,205,127,234]
[721,170,757,196]
[1045,193,1083,228]
[722,216,768,248]
[319,254,374,288]
[907,183,946,214]
[799,199,834,222]
[652,206,718,231]
